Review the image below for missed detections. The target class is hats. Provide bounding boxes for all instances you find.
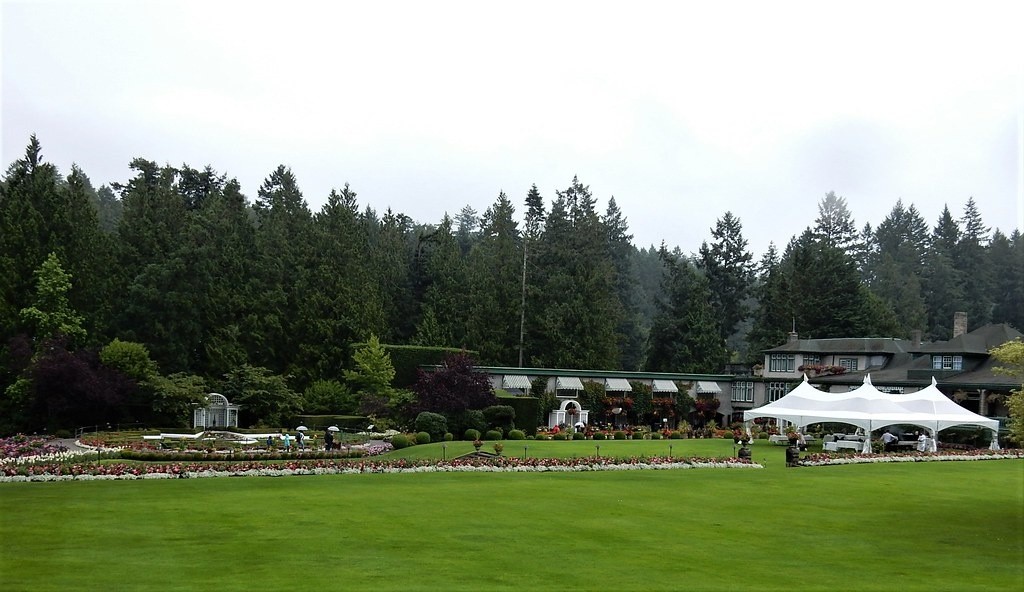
[269,436,272,439]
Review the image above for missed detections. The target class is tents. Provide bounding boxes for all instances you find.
[744,372,999,449]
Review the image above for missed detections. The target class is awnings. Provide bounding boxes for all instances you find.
[503,374,532,390]
[696,380,722,394]
[605,378,632,392]
[556,377,584,391]
[652,379,678,393]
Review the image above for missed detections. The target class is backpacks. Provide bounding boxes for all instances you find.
[295,433,302,442]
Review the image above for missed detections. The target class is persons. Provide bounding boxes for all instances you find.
[917,432,926,452]
[880,430,897,452]
[285,434,290,453]
[324,430,334,452]
[266,436,272,453]
[297,430,304,452]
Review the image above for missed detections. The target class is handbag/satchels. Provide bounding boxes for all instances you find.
[324,443,328,448]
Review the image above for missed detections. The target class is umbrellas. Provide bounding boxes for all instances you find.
[296,426,308,431]
[328,426,340,432]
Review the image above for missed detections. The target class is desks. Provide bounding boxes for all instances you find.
[768,430,938,457]
